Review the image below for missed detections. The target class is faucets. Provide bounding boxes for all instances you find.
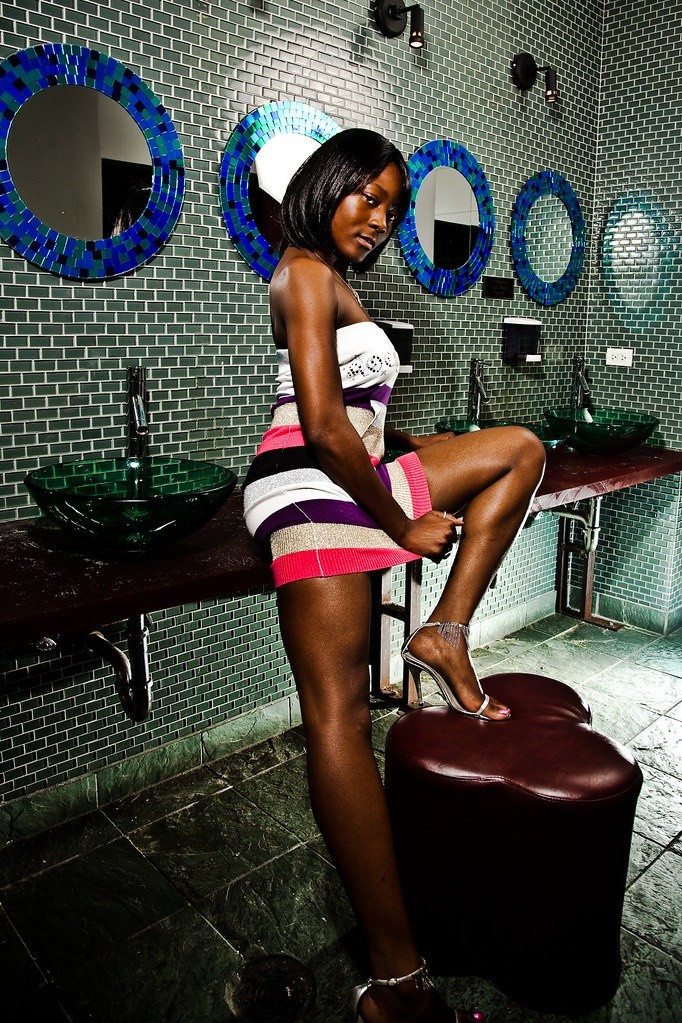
[572,355,591,420]
[125,365,152,496]
[468,358,493,420]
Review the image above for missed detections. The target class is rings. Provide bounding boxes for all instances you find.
[443,511,447,517]
[445,548,451,557]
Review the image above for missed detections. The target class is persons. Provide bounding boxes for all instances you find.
[240,127,548,1023]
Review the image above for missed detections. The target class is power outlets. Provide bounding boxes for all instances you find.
[606,348,633,367]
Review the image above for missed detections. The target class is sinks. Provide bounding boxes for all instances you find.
[434,418,571,460]
[26,454,238,552]
[544,405,660,456]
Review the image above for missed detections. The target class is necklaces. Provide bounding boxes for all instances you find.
[313,251,374,321]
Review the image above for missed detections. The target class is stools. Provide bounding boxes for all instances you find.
[386,674,644,1014]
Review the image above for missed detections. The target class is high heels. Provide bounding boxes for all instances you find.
[349,956,485,1023]
[400,622,511,721]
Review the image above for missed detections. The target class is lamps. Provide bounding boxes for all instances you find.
[509,52,558,103]
[370,0,425,49]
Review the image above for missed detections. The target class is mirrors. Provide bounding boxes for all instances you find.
[0,44,186,279]
[398,139,495,297]
[511,171,585,304]
[220,101,344,282]
